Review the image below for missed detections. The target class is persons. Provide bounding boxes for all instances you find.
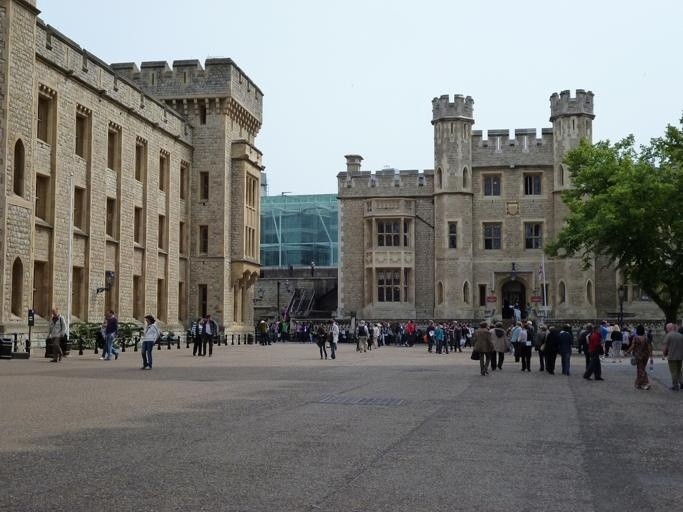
[105,310,117,359]
[473,320,573,375]
[509,301,521,320]
[575,320,637,380]
[426,320,472,355]
[191,317,202,356]
[140,315,158,369]
[625,323,682,390]
[259,312,290,345]
[201,314,217,357]
[291,319,339,360]
[98,311,109,359]
[339,320,421,353]
[47,307,66,361]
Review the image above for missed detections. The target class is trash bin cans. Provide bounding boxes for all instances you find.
[0,338,12,360]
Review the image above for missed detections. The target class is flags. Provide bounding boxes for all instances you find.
[538,255,544,280]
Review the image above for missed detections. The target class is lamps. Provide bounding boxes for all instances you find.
[284,278,292,294]
[249,288,264,303]
[96,271,115,292]
[511,262,515,273]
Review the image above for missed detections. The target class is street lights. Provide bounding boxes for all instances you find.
[617,282,625,329]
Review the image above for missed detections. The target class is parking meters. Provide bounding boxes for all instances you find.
[27,309,34,340]
